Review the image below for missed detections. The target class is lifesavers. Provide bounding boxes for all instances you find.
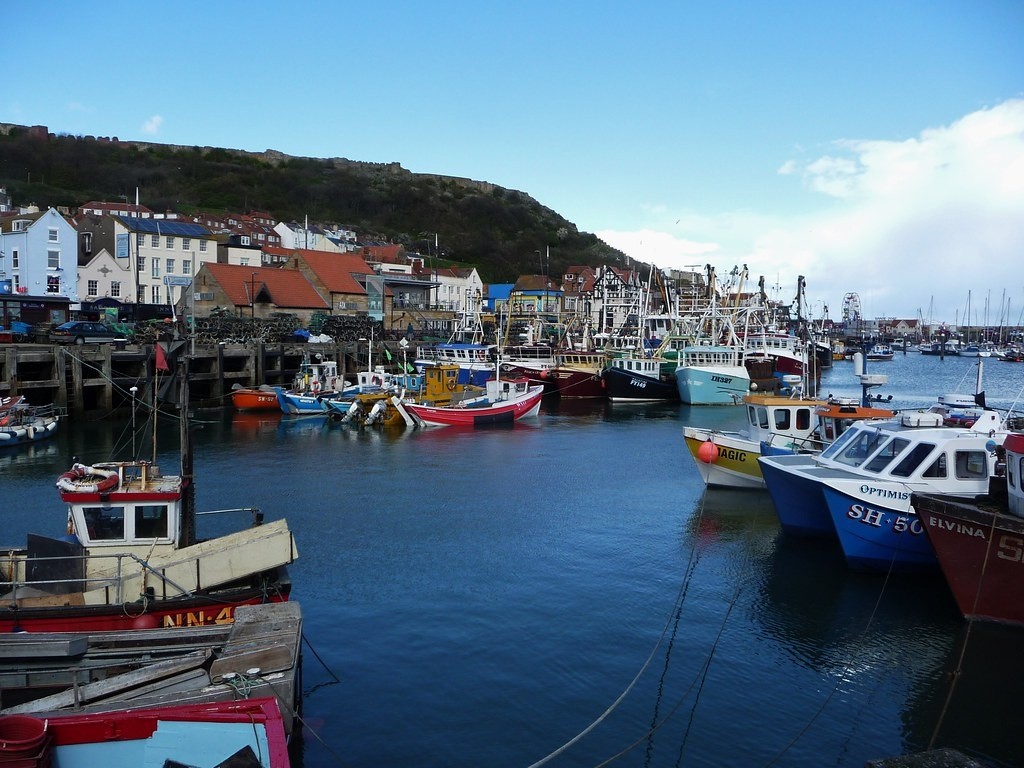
[479,351,486,360]
[311,381,322,393]
[448,379,456,390]
[371,374,382,386]
[55,467,118,493]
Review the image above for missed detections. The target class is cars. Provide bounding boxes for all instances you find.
[49,321,132,345]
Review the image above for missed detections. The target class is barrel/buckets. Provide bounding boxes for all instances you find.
[0,281,12,294]
[0,716,48,760]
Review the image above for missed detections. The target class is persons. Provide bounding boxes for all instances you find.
[407,323,414,341]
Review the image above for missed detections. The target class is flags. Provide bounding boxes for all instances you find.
[398,362,404,369]
[156,344,169,369]
[407,363,414,373]
[385,350,392,360]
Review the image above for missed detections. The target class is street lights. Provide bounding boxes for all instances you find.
[435,232,438,306]
[135,187,140,345]
[250,272,259,317]
[546,244,549,320]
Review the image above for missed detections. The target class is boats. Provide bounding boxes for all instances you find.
[0,360,338,768]
[227,265,904,430]
[682,375,1024,629]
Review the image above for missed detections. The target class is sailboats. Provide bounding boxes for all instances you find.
[918,288,1024,361]
